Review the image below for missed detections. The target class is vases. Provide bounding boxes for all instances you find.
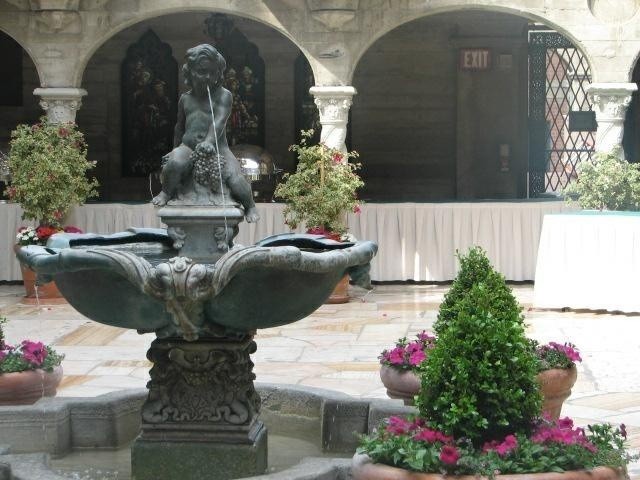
[487,463,629,478]
[380,363,426,406]
[351,451,478,478]
[529,365,577,424]
[13,242,71,305]
[0,364,63,406]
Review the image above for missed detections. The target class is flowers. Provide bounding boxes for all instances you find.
[273,126,367,242]
[351,414,478,479]
[376,328,438,375]
[532,339,584,376]
[0,115,102,247]
[481,411,639,478]
[0,318,66,376]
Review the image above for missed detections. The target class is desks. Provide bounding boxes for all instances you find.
[533,210,640,315]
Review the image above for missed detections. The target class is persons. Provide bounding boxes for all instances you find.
[222,79,251,144]
[149,42,262,224]
[144,80,174,146]
